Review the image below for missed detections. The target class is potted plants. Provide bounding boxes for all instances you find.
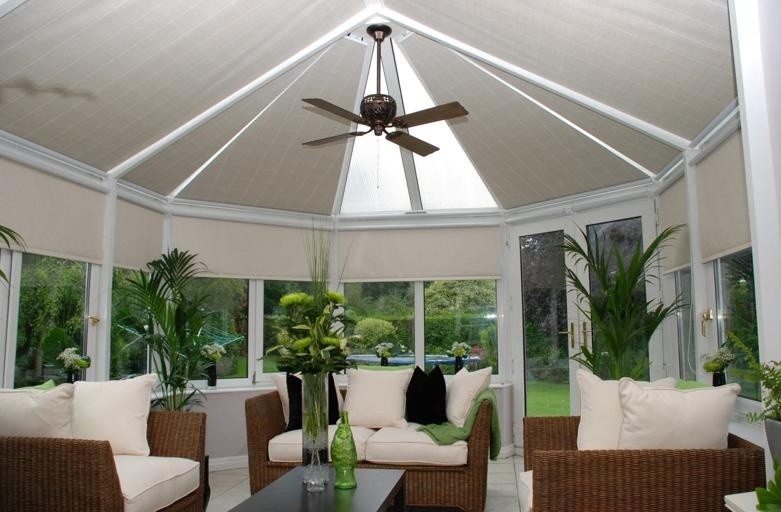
[726,329,781,469]
[117,247,243,511]
[258,219,357,492]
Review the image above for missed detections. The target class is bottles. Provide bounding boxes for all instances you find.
[331,410,360,488]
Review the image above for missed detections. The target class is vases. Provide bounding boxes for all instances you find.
[381,357,388,366]
[455,357,464,374]
[66,370,79,383]
[207,360,216,386]
[712,372,726,387]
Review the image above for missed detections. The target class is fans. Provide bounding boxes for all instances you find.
[302,24,469,158]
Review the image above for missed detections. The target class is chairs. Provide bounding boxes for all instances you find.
[518,417,765,512]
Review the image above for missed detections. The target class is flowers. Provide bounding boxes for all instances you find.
[55,347,91,369]
[375,342,394,357]
[702,344,736,372]
[200,343,227,360]
[447,341,472,357]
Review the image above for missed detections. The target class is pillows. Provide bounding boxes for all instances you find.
[618,377,742,449]
[270,365,493,431]
[73,373,157,455]
[576,367,678,449]
[1,383,74,437]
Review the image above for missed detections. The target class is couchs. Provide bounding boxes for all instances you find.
[245,386,492,511]
[1,411,211,511]
